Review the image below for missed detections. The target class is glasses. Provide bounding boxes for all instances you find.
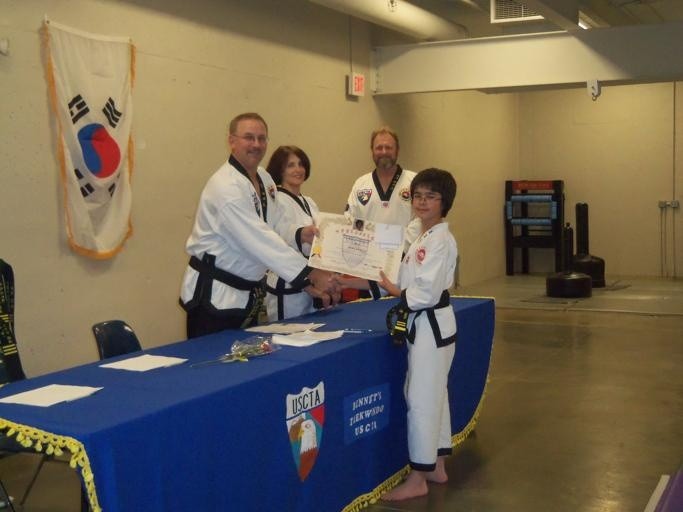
[234,135,270,145]
[414,194,441,202]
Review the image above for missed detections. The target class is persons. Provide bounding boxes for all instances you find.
[352,218,364,232]
[176,114,334,338]
[343,126,419,273]
[264,145,341,323]
[327,167,458,503]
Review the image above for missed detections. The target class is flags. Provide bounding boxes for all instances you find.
[40,15,134,259]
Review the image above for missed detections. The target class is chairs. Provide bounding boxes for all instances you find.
[90,319,143,361]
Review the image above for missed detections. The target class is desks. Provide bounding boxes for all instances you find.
[0,295,496,512]
